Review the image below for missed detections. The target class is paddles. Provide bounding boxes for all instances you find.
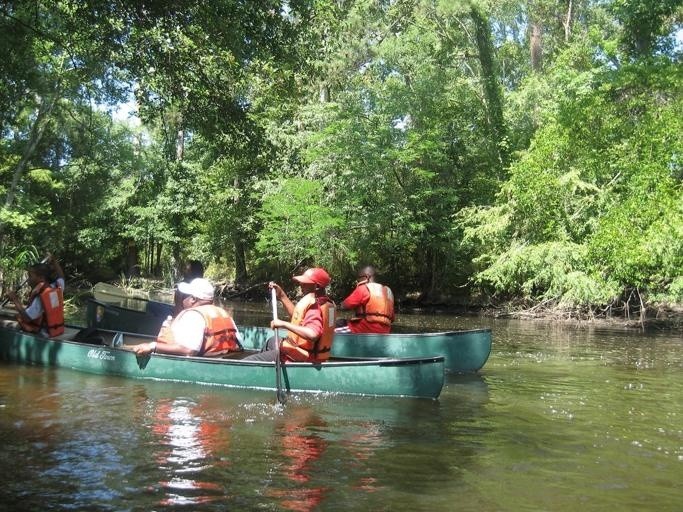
[271,286,286,405]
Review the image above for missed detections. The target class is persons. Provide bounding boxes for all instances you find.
[146,260,204,318]
[131,278,243,358]
[333,265,396,334]
[239,268,338,363]
[4,253,65,339]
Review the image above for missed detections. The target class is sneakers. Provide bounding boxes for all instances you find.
[113,333,123,347]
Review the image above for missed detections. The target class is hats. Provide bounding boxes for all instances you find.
[293,267,330,288]
[178,277,213,300]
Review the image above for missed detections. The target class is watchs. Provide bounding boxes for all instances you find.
[150,341,157,353]
